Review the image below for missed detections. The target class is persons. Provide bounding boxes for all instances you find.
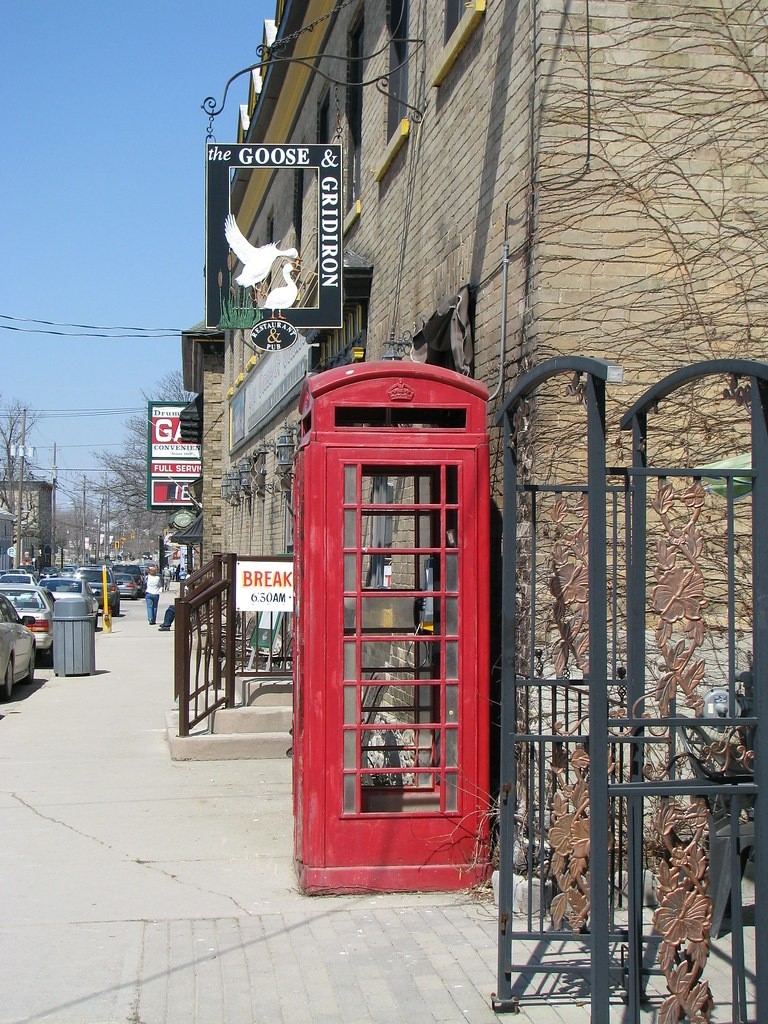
[143,567,162,625]
[158,564,195,631]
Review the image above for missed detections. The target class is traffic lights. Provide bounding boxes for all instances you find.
[115,541,119,549]
[130,533,135,540]
[121,537,126,542]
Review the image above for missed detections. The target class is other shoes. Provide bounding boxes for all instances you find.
[149,620,155,624]
[159,624,170,627]
[158,627,170,631]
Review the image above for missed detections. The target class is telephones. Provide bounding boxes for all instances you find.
[414,558,438,636]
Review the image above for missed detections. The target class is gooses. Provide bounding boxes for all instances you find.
[224,213,304,321]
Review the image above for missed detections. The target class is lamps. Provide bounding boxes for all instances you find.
[219,418,299,500]
[382,328,412,362]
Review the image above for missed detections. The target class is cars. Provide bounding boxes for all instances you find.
[0,574,38,607]
[0,583,56,649]
[30,578,101,629]
[0,551,159,616]
[0,593,36,700]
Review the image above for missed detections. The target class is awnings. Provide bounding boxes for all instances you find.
[171,512,203,544]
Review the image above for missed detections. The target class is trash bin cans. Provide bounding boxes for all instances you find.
[51,597,97,678]
